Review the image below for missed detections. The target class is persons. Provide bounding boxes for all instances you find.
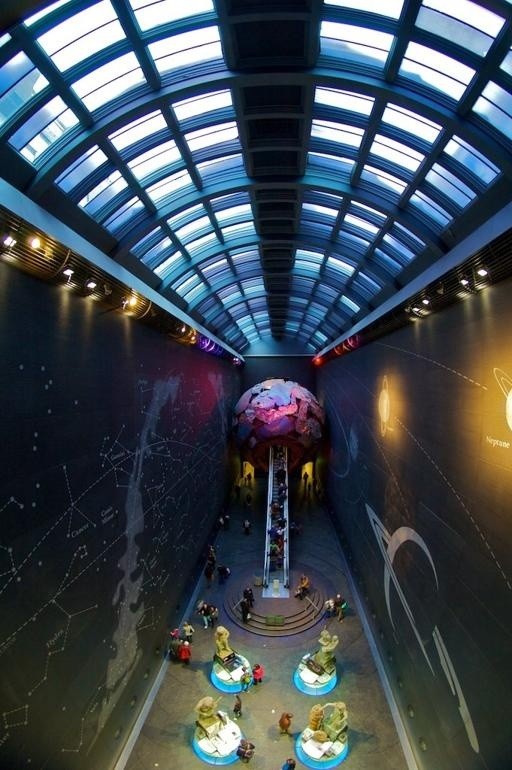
[333,594,346,621]
[232,693,243,721]
[282,759,296,770]
[299,573,311,595]
[279,712,292,734]
[289,471,324,536]
[240,666,253,693]
[251,664,264,685]
[237,739,256,763]
[168,620,195,666]
[324,599,337,618]
[194,445,288,630]
[294,584,304,600]
